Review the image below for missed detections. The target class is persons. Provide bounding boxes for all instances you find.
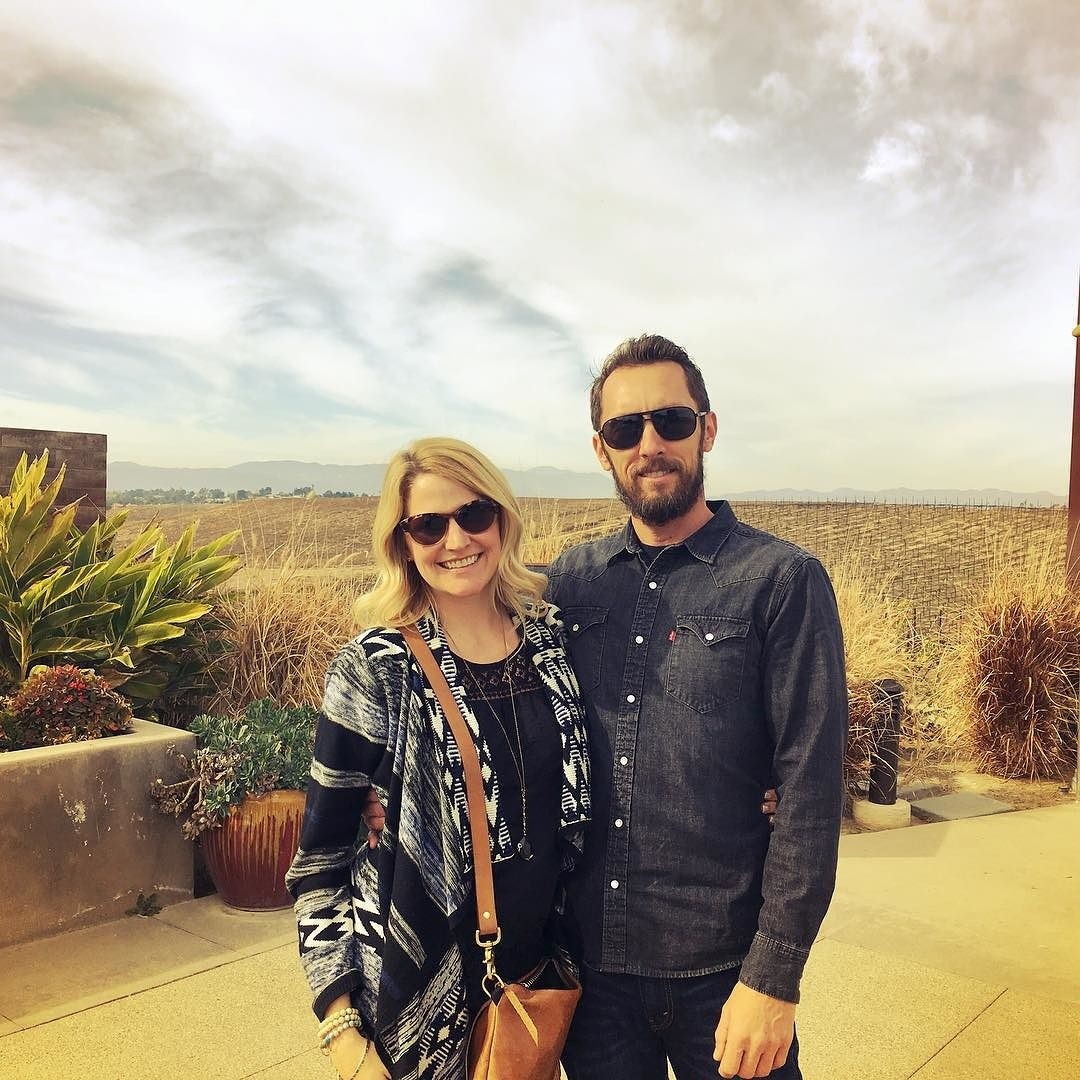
[285,437,778,1080]
[359,336,848,1080]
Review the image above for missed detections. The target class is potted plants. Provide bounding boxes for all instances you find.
[192,697,318,913]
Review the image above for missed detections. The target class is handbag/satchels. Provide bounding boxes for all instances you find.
[466,950,583,1080]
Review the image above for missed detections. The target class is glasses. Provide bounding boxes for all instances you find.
[399,499,501,545]
[599,406,707,450]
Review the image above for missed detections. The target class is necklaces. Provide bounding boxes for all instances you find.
[440,612,534,863]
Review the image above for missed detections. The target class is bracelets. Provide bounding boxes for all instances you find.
[318,1008,362,1048]
[338,1037,370,1080]
